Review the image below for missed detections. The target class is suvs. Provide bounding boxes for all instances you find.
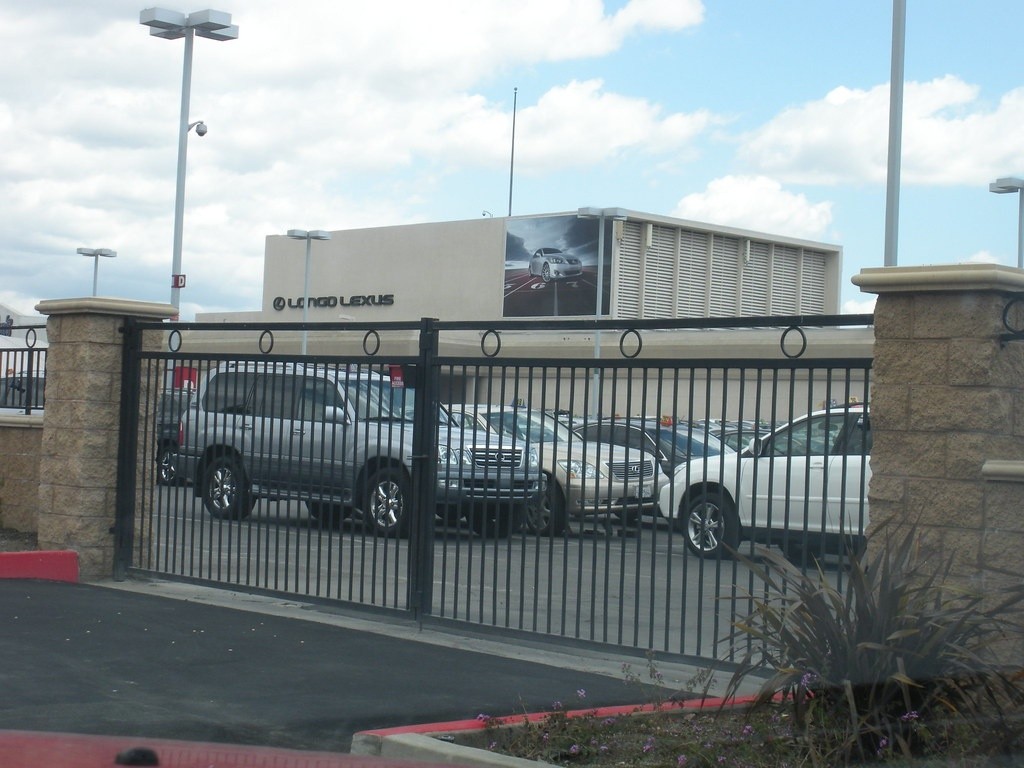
[169,359,548,536]
[660,401,872,559]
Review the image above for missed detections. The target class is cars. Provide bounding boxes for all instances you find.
[391,404,662,538]
[528,248,583,283]
[0,370,202,488]
[549,409,837,481]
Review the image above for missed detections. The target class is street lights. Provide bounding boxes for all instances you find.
[76,247,117,299]
[136,9,240,388]
[286,229,332,355]
[989,176,1024,271]
[482,210,494,219]
